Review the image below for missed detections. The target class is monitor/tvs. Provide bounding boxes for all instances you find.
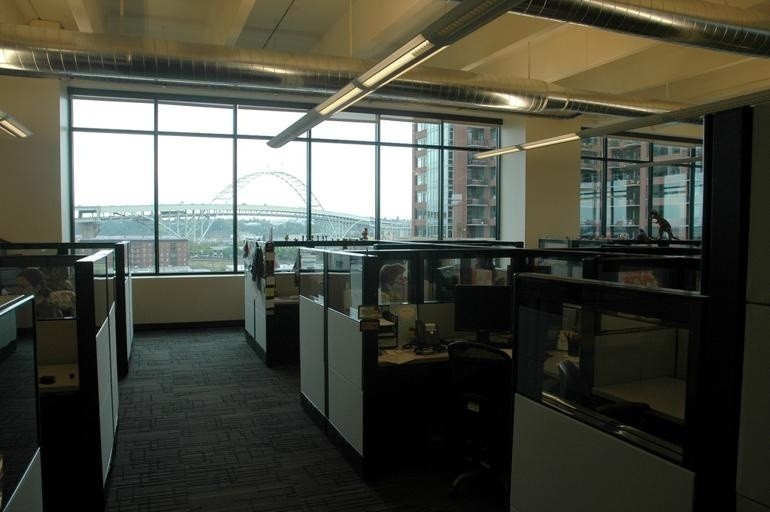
[434,266,458,298]
[453,285,514,349]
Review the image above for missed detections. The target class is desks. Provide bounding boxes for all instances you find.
[274,296,299,305]
[38,363,79,393]
[595,376,685,425]
[378,344,579,381]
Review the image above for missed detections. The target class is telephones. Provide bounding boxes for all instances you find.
[414,320,441,347]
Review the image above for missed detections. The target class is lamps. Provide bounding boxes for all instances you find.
[1,109,35,140]
[472,132,590,159]
[266,27,454,149]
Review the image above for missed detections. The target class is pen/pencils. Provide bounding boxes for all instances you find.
[564,332,581,341]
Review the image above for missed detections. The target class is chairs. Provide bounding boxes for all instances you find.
[448,340,512,497]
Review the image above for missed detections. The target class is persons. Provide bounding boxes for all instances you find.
[376,263,407,305]
[15,268,66,318]
